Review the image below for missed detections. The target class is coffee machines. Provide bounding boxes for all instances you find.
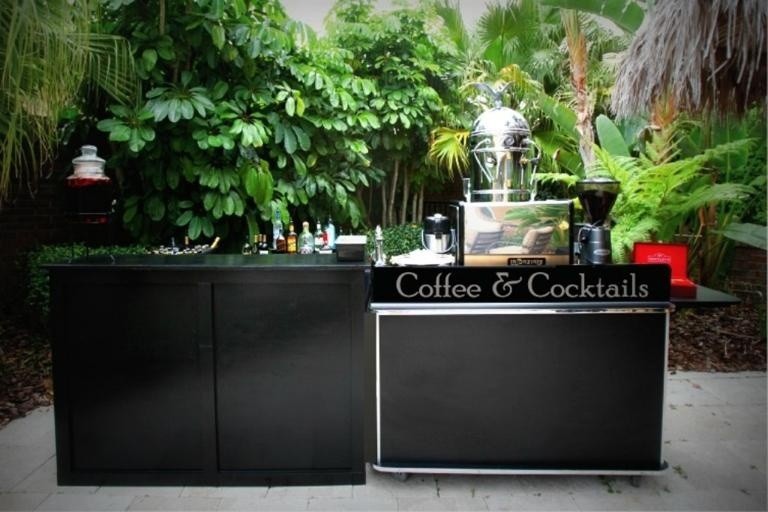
[576,177,621,265]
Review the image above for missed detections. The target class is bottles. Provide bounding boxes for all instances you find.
[297,221,314,255]
[287,219,298,254]
[272,208,284,249]
[324,216,337,250]
[314,219,328,252]
[276,227,287,254]
[64,144,112,214]
[239,233,270,257]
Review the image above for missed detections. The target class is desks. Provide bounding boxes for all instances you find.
[41,252,741,484]
